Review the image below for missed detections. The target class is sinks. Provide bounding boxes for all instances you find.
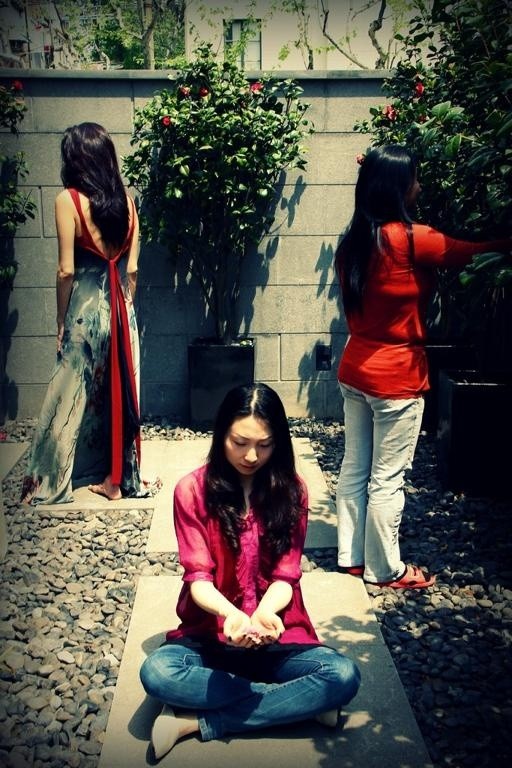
[423,342,511,383]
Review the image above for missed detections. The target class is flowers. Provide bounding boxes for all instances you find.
[123,40,318,342]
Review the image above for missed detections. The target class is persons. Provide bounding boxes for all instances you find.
[139,383,362,766]
[336,142,503,590]
[22,121,150,506]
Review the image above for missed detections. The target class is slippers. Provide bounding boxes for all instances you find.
[346,567,364,575]
[374,563,436,588]
[314,705,344,731]
[146,704,178,765]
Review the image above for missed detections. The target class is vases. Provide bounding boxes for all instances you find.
[187,333,255,432]
[424,337,511,495]
